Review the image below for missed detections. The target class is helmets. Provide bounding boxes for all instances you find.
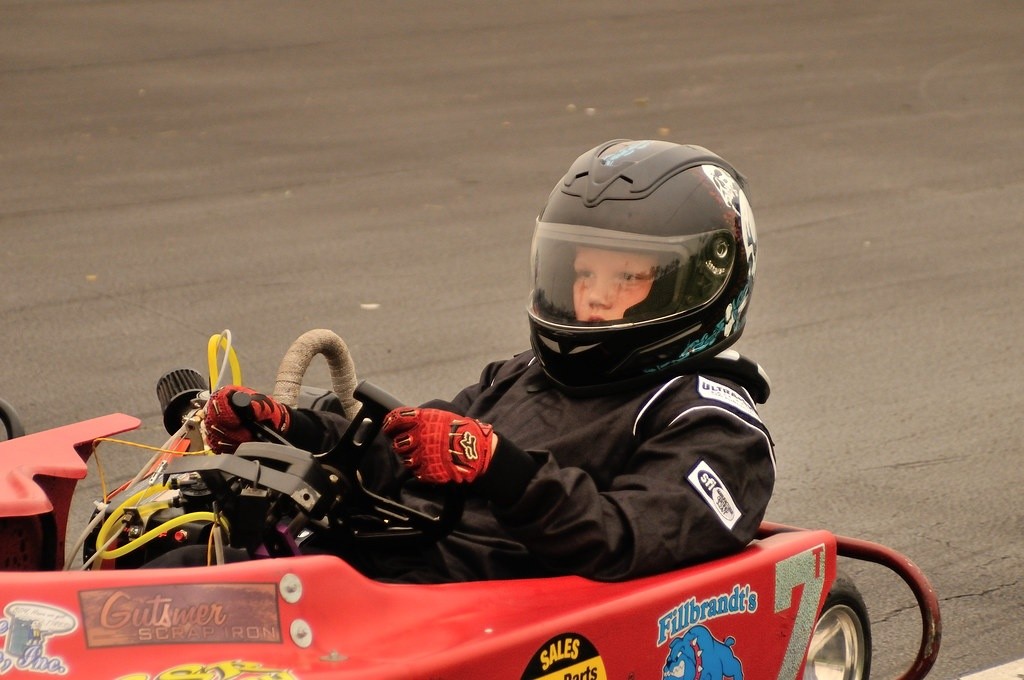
[525,138,758,401]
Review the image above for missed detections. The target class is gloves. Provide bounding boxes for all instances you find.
[203,383,294,456]
[381,406,494,485]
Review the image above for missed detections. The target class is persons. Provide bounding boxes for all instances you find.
[93,139,777,591]
